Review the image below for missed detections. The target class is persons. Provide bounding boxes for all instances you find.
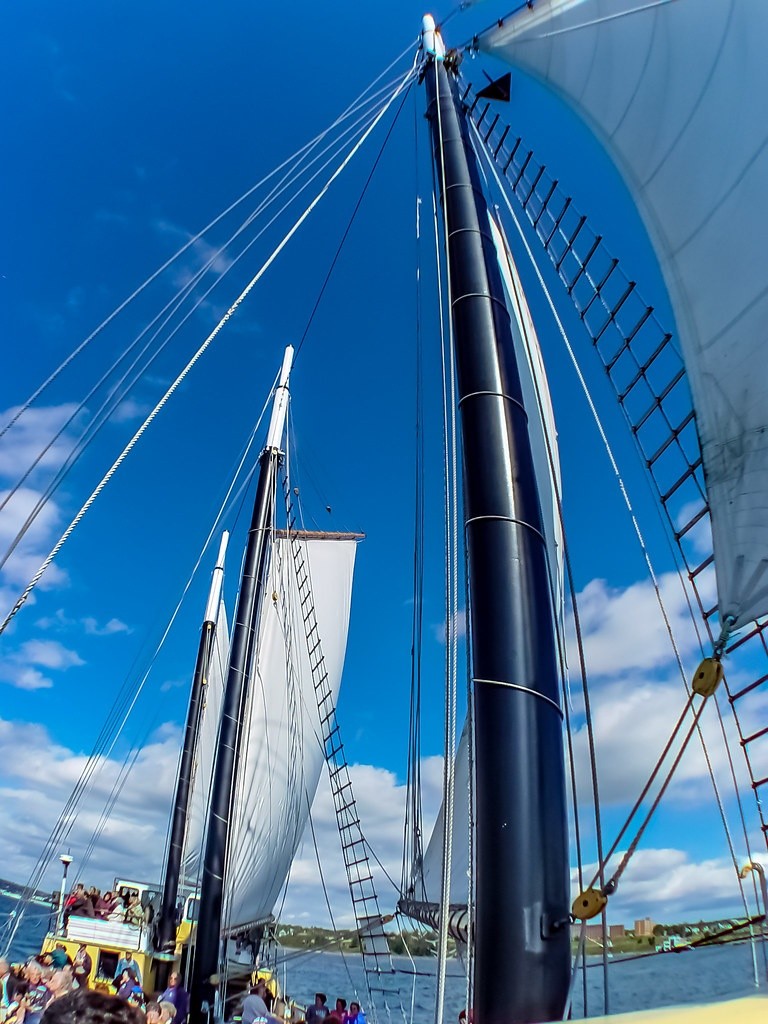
[0,944,191,1024]
[65,884,145,924]
[241,978,365,1024]
